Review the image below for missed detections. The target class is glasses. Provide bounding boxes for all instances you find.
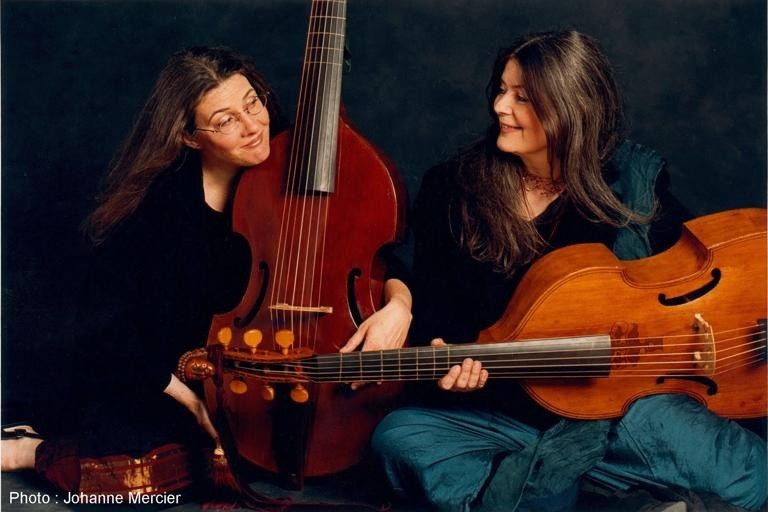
[194,91,271,134]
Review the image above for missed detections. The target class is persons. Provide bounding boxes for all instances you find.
[369,31,768,512]
[0,49,413,512]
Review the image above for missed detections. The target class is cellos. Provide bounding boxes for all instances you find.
[176,208,766,421]
[204,3,409,481]
